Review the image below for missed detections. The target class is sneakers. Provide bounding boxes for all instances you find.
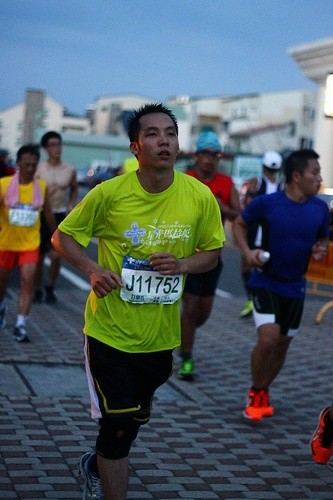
[0,305,8,329]
[79,451,107,499]
[13,326,29,342]
[240,301,254,320]
[242,388,263,419]
[44,285,58,304]
[261,390,273,416]
[31,288,42,306]
[175,352,195,380]
[310,406,333,464]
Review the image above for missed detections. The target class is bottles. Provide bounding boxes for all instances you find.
[253,251,269,264]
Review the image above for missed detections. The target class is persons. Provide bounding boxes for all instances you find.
[236,150,333,422]
[1,150,16,179]
[50,102,226,500]
[0,143,59,342]
[177,130,241,380]
[232,149,285,317]
[309,405,333,465]
[29,130,80,305]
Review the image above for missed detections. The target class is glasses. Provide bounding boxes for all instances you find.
[199,150,223,159]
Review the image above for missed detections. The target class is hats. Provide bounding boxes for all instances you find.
[262,150,284,170]
[196,131,221,150]
